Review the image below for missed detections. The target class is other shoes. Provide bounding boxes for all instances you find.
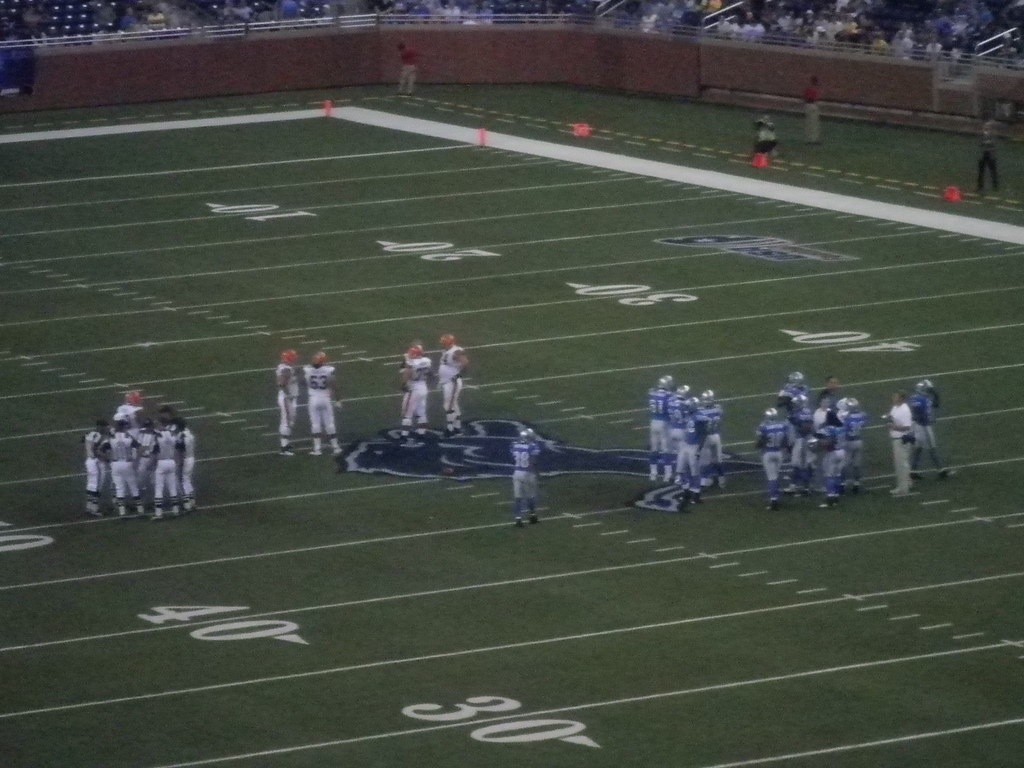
[783,484,796,493]
[279,451,294,456]
[889,485,910,495]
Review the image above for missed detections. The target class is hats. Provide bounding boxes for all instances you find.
[752,115,775,130]
[97,418,109,426]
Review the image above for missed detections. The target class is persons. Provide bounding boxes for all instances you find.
[275,348,344,456]
[80,391,196,520]
[646,371,956,512]
[509,428,547,527]
[751,112,776,153]
[396,40,428,96]
[0,0,1024,71]
[974,110,1002,191]
[397,334,470,446]
[802,74,825,145]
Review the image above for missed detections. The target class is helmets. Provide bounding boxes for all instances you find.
[128,392,143,406]
[281,350,297,364]
[520,428,535,441]
[763,371,861,420]
[915,379,935,389]
[313,352,327,365]
[439,334,454,347]
[659,374,715,407]
[408,345,424,358]
